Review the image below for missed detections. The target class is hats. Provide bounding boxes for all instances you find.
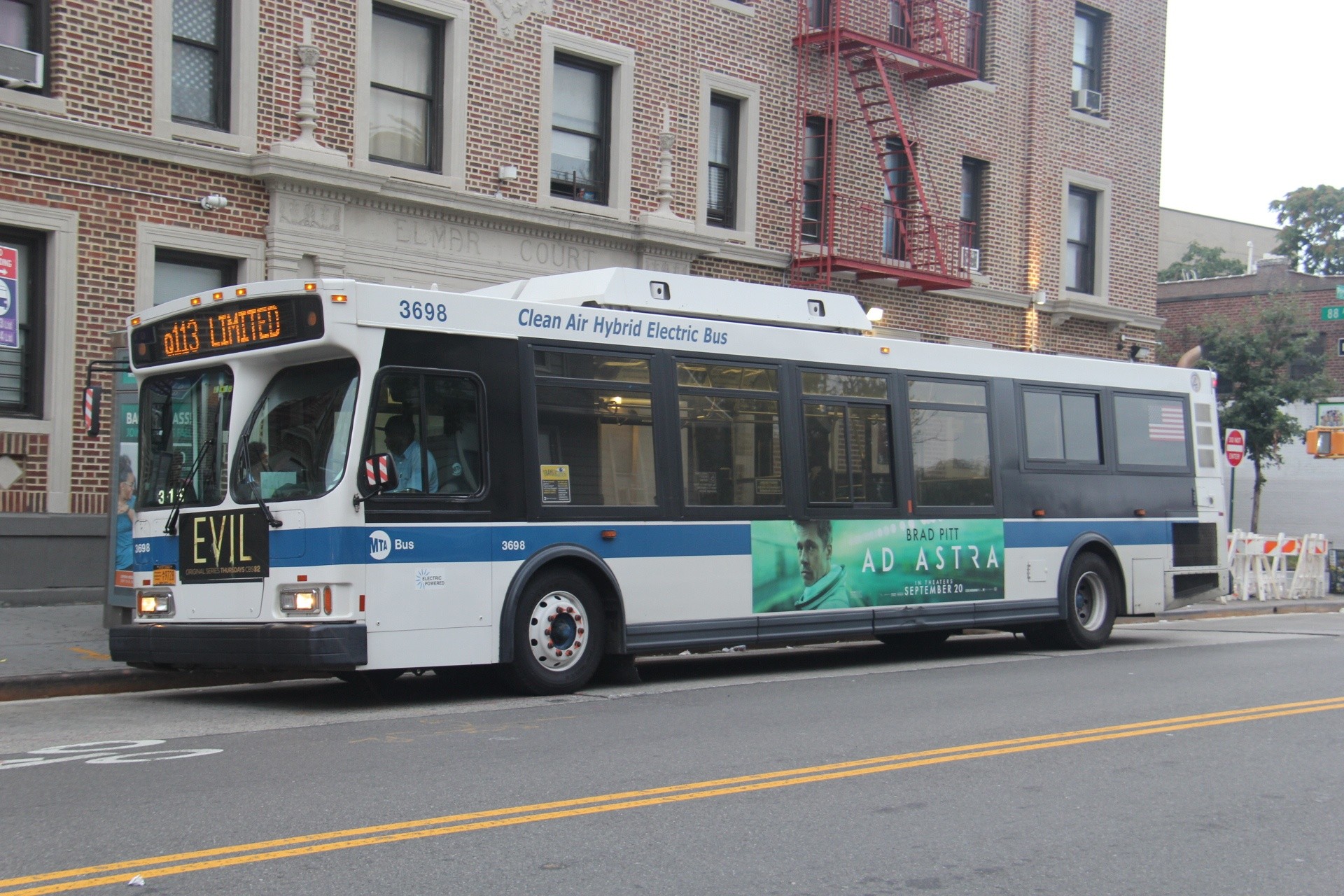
[239,441,269,469]
[376,415,415,433]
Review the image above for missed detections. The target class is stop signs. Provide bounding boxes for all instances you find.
[1225,430,1245,468]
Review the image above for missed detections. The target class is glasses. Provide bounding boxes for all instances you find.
[123,482,137,489]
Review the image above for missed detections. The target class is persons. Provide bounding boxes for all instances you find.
[793,519,855,612]
[116,457,137,595]
[383,415,440,495]
[236,440,271,499]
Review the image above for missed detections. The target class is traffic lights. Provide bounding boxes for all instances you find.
[1307,429,1332,455]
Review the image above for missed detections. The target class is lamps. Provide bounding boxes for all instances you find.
[1032,291,1045,305]
[201,194,228,210]
[499,166,517,179]
[1131,346,1149,359]
[865,305,884,321]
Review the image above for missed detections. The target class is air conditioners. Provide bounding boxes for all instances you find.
[960,246,979,270]
[0,44,44,89]
[1072,88,1102,114]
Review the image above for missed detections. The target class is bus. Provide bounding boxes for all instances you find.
[83,265,1229,696]
